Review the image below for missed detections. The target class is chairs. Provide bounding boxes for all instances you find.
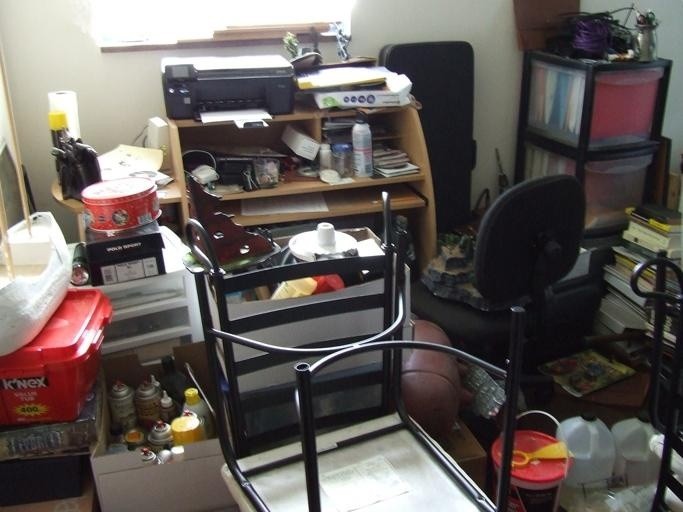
[181,174,683,512]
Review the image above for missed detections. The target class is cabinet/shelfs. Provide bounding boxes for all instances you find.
[510,50,672,244]
[64,224,206,366]
[50,97,439,283]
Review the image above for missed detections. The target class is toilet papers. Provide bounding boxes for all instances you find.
[47,89,81,141]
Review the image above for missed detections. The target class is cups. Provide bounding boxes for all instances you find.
[254,158,280,187]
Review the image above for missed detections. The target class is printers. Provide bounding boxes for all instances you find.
[161,57,295,130]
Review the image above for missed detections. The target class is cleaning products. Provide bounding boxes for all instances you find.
[351,109,374,179]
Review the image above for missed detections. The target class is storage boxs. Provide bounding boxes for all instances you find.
[85,338,238,511]
[0,288,114,429]
[83,221,167,288]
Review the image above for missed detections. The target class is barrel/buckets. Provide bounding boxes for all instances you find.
[377,214,418,293]
[610,408,663,488]
[491,408,573,512]
[555,409,615,512]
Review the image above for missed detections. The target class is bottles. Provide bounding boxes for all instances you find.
[333,145,352,178]
[47,110,70,151]
[352,116,375,177]
[107,353,212,462]
[638,29,649,62]
[319,145,331,171]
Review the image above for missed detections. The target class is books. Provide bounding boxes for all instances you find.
[591,204,683,383]
